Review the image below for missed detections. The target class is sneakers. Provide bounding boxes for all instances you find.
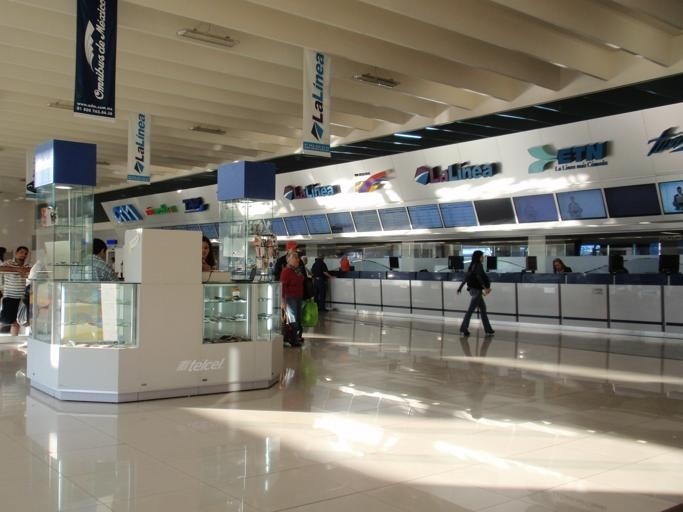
[285,336,305,348]
[459,328,471,336]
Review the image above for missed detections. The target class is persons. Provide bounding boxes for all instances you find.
[670,186,683,210]
[454,249,496,336]
[299,255,312,278]
[0,246,52,338]
[457,333,494,421]
[279,349,316,452]
[71,238,118,282]
[310,252,335,312]
[276,252,305,348]
[550,259,572,275]
[34,204,55,226]
[565,194,584,218]
[201,236,219,270]
[273,239,310,343]
[312,310,328,355]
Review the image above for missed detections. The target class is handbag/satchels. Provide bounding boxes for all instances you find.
[301,299,319,327]
[465,263,484,291]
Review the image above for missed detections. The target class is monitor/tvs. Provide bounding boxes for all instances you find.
[525,255,538,273]
[659,255,679,274]
[486,255,498,270]
[388,257,399,269]
[448,255,464,271]
[608,254,625,274]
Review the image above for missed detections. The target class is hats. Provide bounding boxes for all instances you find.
[287,240,297,251]
